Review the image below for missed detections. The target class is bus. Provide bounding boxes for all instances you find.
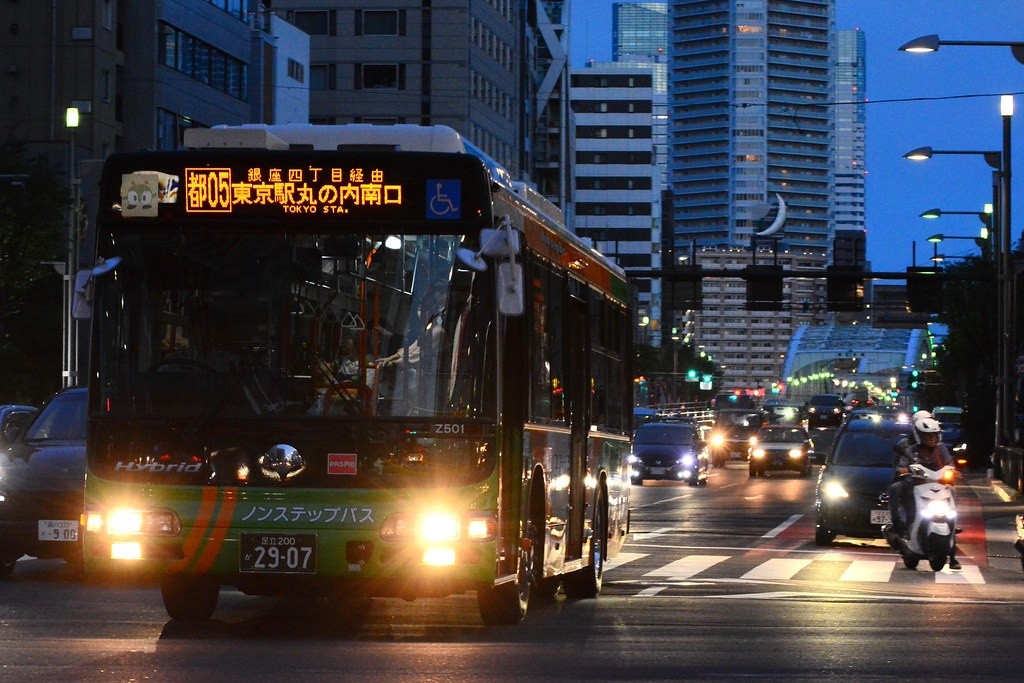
[72,124,639,629]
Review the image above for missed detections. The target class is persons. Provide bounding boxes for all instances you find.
[325,339,374,392]
[163,302,240,374]
[373,300,451,417]
[889,410,962,569]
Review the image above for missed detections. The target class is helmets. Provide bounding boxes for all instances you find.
[912,410,932,426]
[914,417,941,445]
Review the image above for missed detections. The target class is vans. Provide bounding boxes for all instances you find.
[931,405,966,443]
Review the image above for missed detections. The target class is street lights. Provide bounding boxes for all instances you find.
[903,93,1015,482]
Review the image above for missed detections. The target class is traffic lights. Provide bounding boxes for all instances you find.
[702,373,713,385]
[687,370,700,380]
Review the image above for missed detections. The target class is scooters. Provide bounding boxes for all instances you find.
[882,442,967,572]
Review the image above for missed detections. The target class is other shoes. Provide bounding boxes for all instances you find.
[898,528,910,540]
[887,523,903,532]
[954,528,962,534]
[949,561,961,569]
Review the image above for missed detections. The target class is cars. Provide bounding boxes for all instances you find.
[809,413,911,547]
[0,404,42,449]
[711,393,845,467]
[629,421,712,488]
[749,425,815,477]
[0,383,90,575]
